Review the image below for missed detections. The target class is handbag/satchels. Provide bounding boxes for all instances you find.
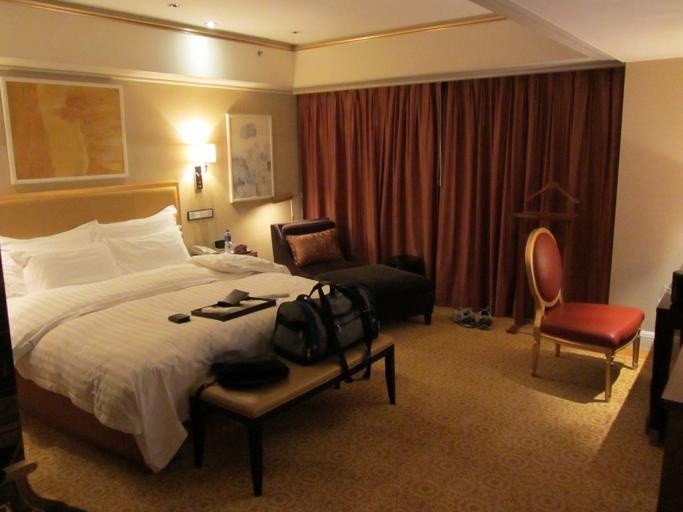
[209,349,290,391]
[272,281,380,366]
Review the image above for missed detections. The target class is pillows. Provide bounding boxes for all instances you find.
[0,206,191,294]
[285,226,344,267]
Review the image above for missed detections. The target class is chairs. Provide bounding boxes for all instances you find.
[519,224,645,403]
[266,213,435,337]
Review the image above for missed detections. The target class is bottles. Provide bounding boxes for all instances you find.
[224,229,232,254]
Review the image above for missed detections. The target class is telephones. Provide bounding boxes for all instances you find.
[191,245,217,255]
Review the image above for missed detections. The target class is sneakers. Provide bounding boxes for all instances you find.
[478,309,493,330]
[451,308,477,328]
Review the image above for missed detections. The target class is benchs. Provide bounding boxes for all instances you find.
[187,329,401,494]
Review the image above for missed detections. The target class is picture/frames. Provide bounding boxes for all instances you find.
[225,111,276,204]
[0,76,127,185]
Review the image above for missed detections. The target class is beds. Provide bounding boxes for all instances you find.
[0,177,333,470]
